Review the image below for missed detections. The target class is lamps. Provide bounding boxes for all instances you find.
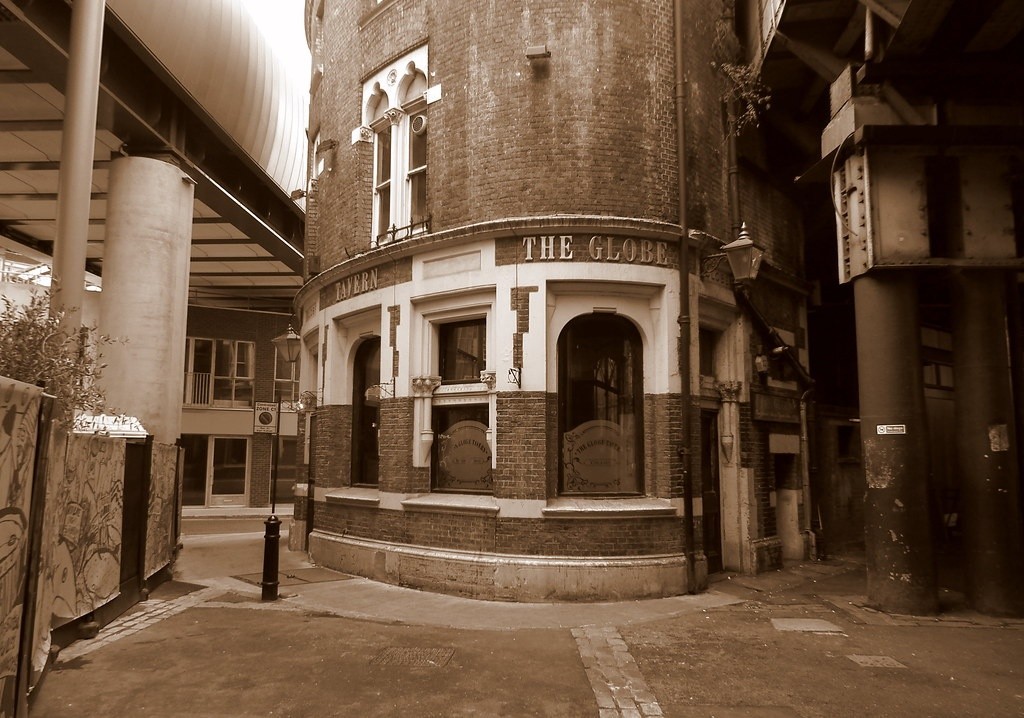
[699,222,766,290]
[288,189,306,201]
[270,324,304,363]
